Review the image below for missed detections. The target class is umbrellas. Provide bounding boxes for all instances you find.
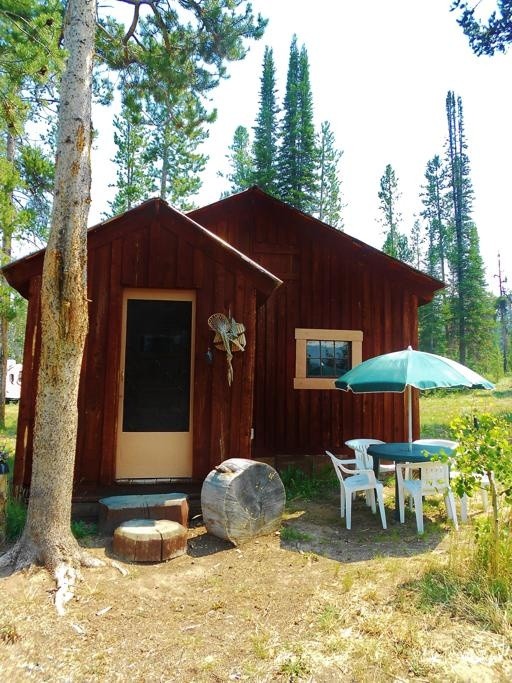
[332,342,496,510]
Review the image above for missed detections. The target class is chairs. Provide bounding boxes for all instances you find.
[325,437,489,534]
